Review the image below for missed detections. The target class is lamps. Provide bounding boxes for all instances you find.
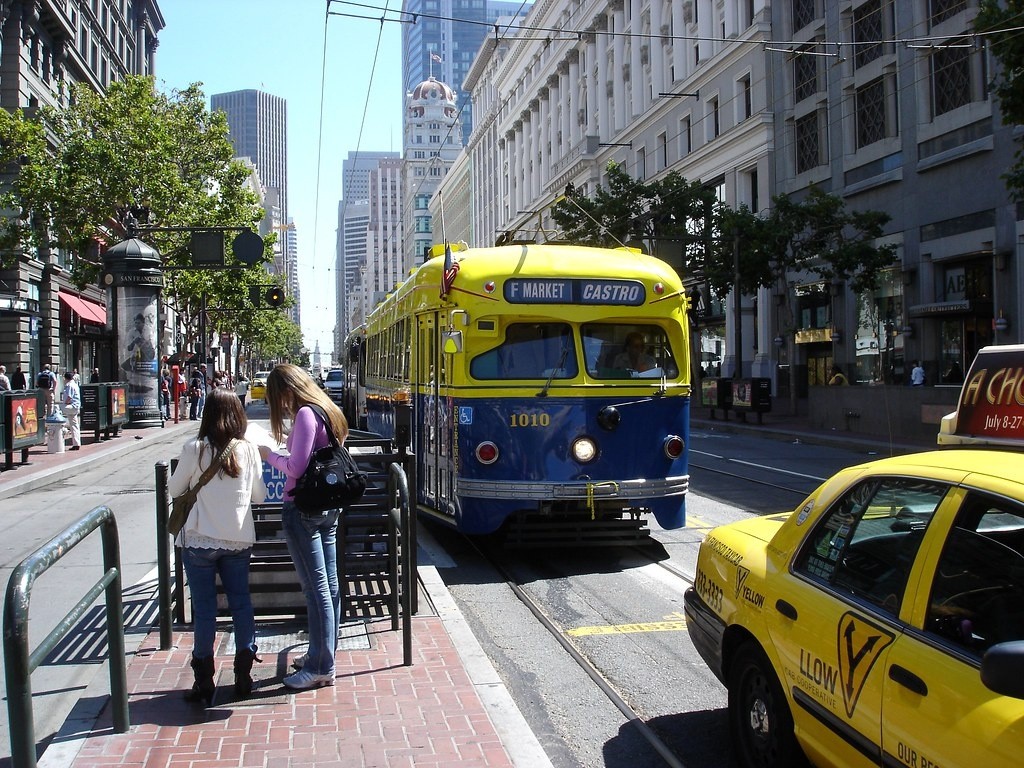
[774,337,782,346]
[995,318,1007,330]
[830,333,839,342]
[903,327,912,337]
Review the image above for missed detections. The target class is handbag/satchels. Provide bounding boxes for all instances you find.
[163,486,197,538]
[282,402,370,525]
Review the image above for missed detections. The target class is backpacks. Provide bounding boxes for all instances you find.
[38,372,51,389]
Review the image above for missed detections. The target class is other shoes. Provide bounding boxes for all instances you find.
[69,446,80,450]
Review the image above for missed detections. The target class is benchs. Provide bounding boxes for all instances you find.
[842,529,1024,589]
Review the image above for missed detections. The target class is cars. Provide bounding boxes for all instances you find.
[324,365,344,405]
[255,371,270,378]
[683,344,1024,768]
[307,368,327,389]
[250,378,269,405]
[348,374,356,387]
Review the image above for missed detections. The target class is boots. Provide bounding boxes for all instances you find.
[232,651,257,699]
[182,651,216,707]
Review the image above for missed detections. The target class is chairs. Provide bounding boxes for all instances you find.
[930,584,1023,649]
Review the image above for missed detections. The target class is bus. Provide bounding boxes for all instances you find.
[346,245,691,552]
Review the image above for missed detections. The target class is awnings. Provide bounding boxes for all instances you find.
[57,291,106,325]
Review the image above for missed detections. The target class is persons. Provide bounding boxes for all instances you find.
[0,365,10,391]
[58,372,81,450]
[167,387,267,708]
[37,363,100,420]
[160,363,256,421]
[700,361,721,377]
[613,332,657,373]
[257,363,350,690]
[11,365,26,390]
[910,361,926,388]
[500,323,546,377]
[120,312,156,371]
[829,365,851,386]
[950,361,962,383]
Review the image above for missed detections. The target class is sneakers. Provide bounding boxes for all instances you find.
[282,652,339,691]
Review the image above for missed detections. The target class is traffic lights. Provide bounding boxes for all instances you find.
[265,288,284,306]
